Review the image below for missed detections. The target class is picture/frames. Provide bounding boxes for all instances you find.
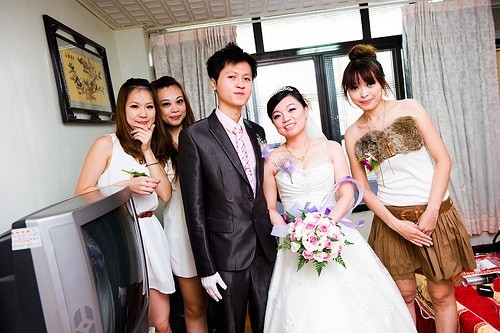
[43,14,117,123]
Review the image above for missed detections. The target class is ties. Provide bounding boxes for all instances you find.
[233,124,256,201]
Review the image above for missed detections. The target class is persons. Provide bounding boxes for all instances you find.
[262,86,418,333]
[178,42,282,333]
[75,78,176,333]
[342,45,476,333]
[149,76,209,333]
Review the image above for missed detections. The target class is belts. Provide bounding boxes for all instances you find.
[136,211,153,218]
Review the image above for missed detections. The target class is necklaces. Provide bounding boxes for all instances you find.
[362,100,386,136]
[284,138,310,161]
[138,157,144,165]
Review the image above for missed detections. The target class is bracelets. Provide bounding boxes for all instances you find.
[145,162,160,167]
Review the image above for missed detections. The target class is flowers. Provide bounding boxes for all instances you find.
[256,132,269,160]
[277,155,296,185]
[357,151,379,172]
[121,170,148,178]
[276,208,355,278]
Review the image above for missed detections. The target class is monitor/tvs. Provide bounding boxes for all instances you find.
[0,184,150,333]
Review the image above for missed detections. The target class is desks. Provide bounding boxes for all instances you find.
[412,253,500,333]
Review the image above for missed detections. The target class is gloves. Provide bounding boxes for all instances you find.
[201,273,227,302]
